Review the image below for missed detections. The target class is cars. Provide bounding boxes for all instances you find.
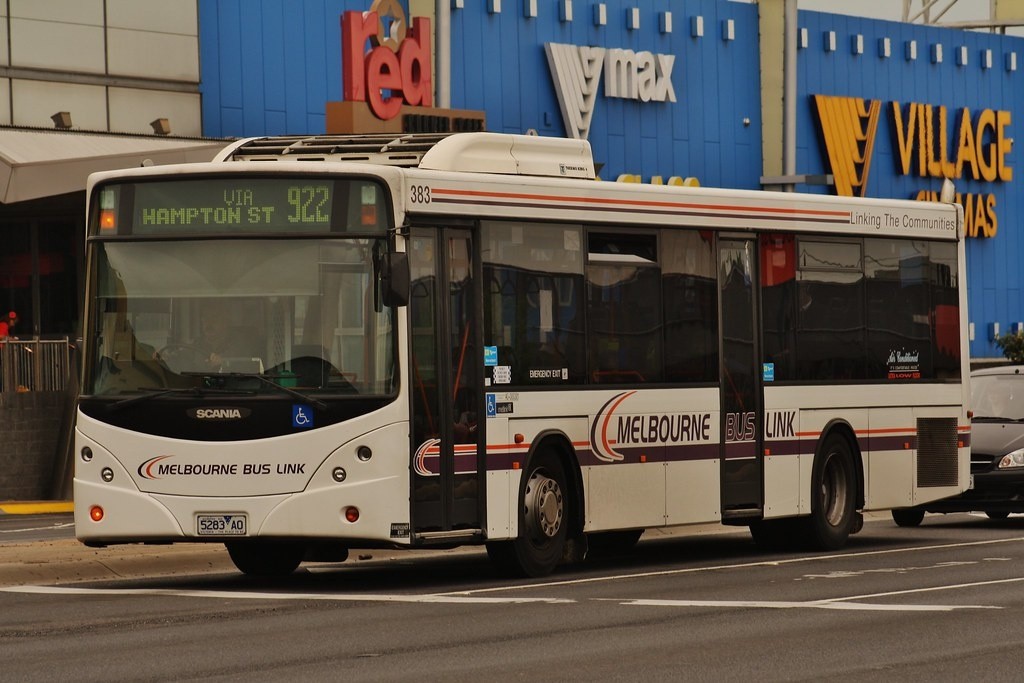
[893,366,1024,528]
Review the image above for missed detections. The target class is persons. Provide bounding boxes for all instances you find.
[153,300,266,381]
[0,311,18,348]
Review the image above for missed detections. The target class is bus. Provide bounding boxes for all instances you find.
[74,132,973,580]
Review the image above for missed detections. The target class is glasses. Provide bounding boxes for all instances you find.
[201,315,219,323]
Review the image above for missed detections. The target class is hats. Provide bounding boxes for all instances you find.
[4,311,17,321]
